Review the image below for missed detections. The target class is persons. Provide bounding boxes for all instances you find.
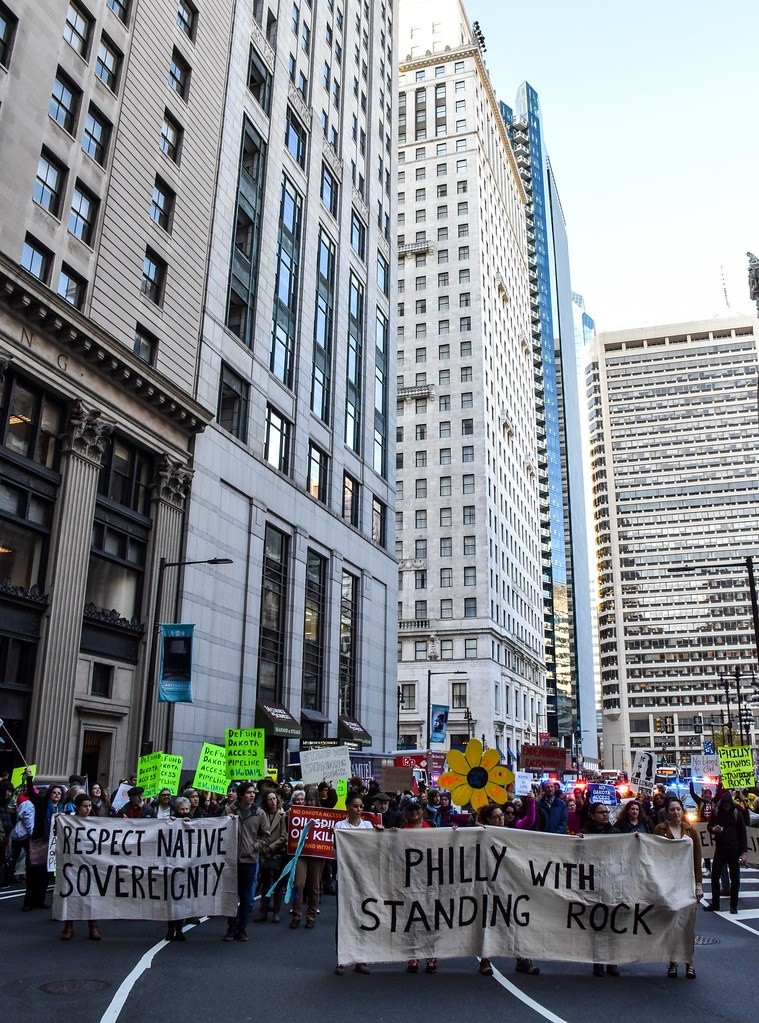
[0,762,759,974]
[433,713,444,731]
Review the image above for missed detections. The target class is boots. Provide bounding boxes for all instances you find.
[166,916,187,943]
[60,918,103,941]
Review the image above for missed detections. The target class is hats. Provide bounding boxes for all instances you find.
[402,795,424,810]
[127,787,145,796]
[370,792,391,802]
[426,787,451,800]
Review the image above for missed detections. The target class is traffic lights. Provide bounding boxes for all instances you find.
[656,716,664,733]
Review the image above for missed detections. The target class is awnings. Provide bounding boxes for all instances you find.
[339,713,373,748]
[254,698,302,739]
[300,708,332,725]
[507,748,516,761]
[496,747,506,760]
[517,750,521,757]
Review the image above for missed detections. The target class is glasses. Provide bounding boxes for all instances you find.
[489,811,516,820]
[568,804,577,807]
[161,794,171,797]
[593,810,611,815]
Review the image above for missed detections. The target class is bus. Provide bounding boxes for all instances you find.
[654,767,683,786]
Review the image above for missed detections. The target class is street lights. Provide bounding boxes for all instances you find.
[536,713,569,746]
[397,686,405,742]
[464,707,472,742]
[140,557,234,755]
[612,744,626,769]
[575,731,590,780]
[427,670,467,748]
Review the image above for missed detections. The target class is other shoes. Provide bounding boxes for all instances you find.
[667,963,696,978]
[703,871,711,878]
[224,927,251,941]
[479,959,494,974]
[702,904,739,914]
[20,902,51,912]
[720,889,730,896]
[409,959,438,973]
[257,910,279,923]
[335,963,372,975]
[593,964,619,978]
[0,875,26,891]
[289,907,320,929]
[515,959,541,975]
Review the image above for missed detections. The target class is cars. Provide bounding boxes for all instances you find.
[665,778,718,823]
